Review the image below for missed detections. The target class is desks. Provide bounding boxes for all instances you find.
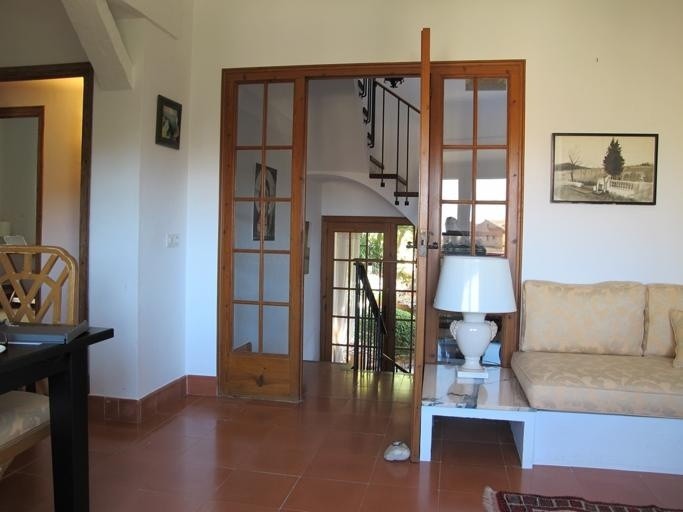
[0,322,112,510]
[419,364,534,469]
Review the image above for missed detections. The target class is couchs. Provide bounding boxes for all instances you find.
[512,282,682,473]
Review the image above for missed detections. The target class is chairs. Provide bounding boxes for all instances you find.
[0,245,77,476]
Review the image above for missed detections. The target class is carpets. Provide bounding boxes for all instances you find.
[482,487,682,512]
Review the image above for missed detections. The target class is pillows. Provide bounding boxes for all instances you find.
[668,307,683,369]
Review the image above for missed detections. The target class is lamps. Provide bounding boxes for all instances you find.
[432,255,517,379]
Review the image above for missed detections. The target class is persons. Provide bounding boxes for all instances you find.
[256,180,271,238]
[442,217,486,255]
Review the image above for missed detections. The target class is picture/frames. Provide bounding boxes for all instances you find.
[153,94,181,151]
[551,133,658,204]
